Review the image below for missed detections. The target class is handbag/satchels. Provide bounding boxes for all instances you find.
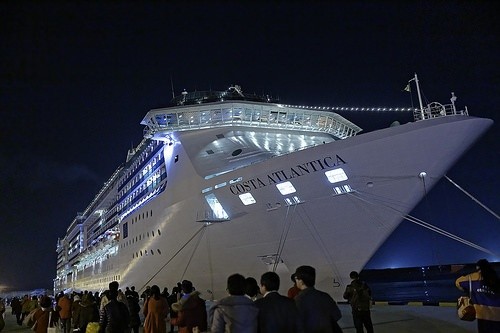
[456,274,475,321]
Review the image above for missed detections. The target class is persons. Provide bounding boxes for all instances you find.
[0,296,5,333]
[253,272,297,333]
[99,282,131,328]
[343,271,374,333]
[58,295,73,333]
[244,277,263,301]
[112,279,188,333]
[204,289,218,333]
[293,265,344,333]
[210,274,261,333]
[288,273,301,299]
[128,297,141,333]
[142,285,169,333]
[7,290,101,328]
[72,294,100,333]
[33,303,54,333]
[169,281,208,333]
[167,287,185,333]
[456,259,500,333]
[100,290,131,333]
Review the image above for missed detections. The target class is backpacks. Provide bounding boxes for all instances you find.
[350,282,371,311]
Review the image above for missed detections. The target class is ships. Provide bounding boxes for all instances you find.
[53,74,495,303]
